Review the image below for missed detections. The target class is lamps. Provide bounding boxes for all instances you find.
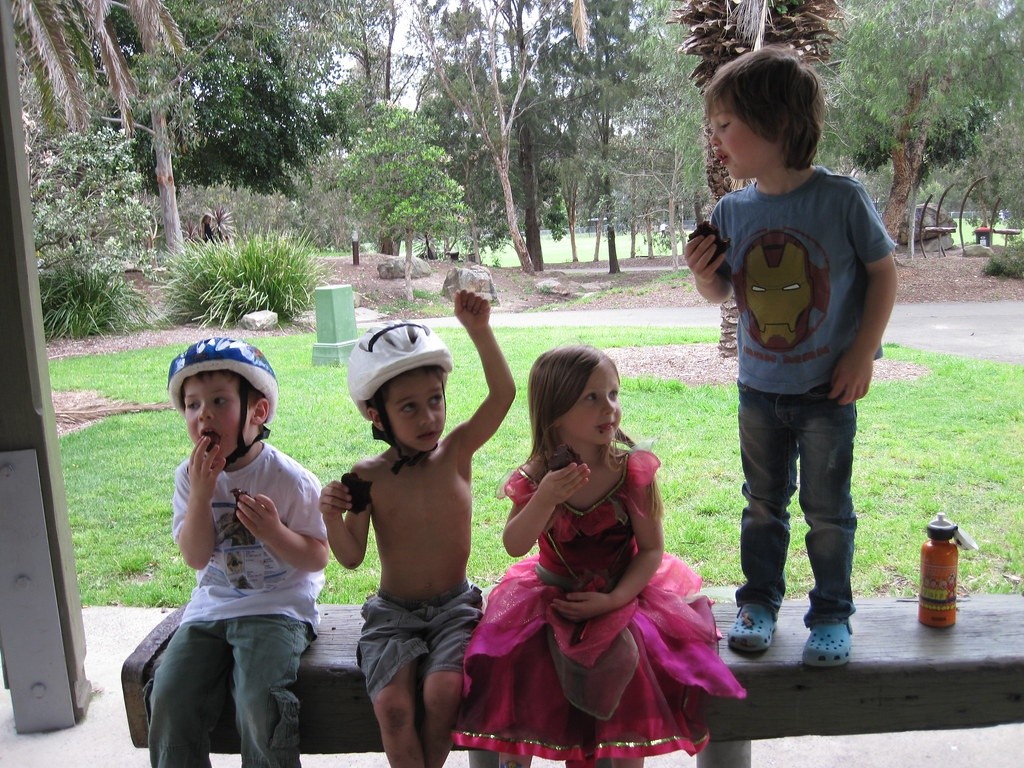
[351,231,359,240]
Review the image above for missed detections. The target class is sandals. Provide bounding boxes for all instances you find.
[803,624,853,666]
[728,602,777,652]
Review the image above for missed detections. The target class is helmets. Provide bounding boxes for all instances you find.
[347,319,454,421]
[167,337,279,423]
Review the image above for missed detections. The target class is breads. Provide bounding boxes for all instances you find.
[688,220,730,264]
[233,488,255,523]
[340,472,374,514]
[548,444,582,471]
[202,431,217,451]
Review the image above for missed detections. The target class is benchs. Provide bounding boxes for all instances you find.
[119,594,1024,768]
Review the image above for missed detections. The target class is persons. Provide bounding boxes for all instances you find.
[141,337,327,768]
[683,48,895,667]
[451,345,747,768]
[320,289,516,768]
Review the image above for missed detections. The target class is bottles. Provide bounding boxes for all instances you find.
[917,512,979,627]
